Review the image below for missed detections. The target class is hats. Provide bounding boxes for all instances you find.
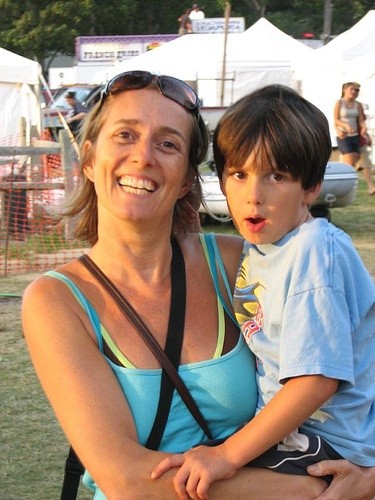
[64,92,76,98]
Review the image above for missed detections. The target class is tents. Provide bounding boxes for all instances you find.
[1,10,374,173]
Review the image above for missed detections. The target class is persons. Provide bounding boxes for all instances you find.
[63,91,86,132]
[151,84,374,500]
[334,81,374,196]
[21,69,375,500]
[177,4,205,34]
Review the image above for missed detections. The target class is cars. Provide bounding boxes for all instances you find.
[42,83,106,128]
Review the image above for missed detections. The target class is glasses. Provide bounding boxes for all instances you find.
[99,70,201,113]
[355,89,360,92]
